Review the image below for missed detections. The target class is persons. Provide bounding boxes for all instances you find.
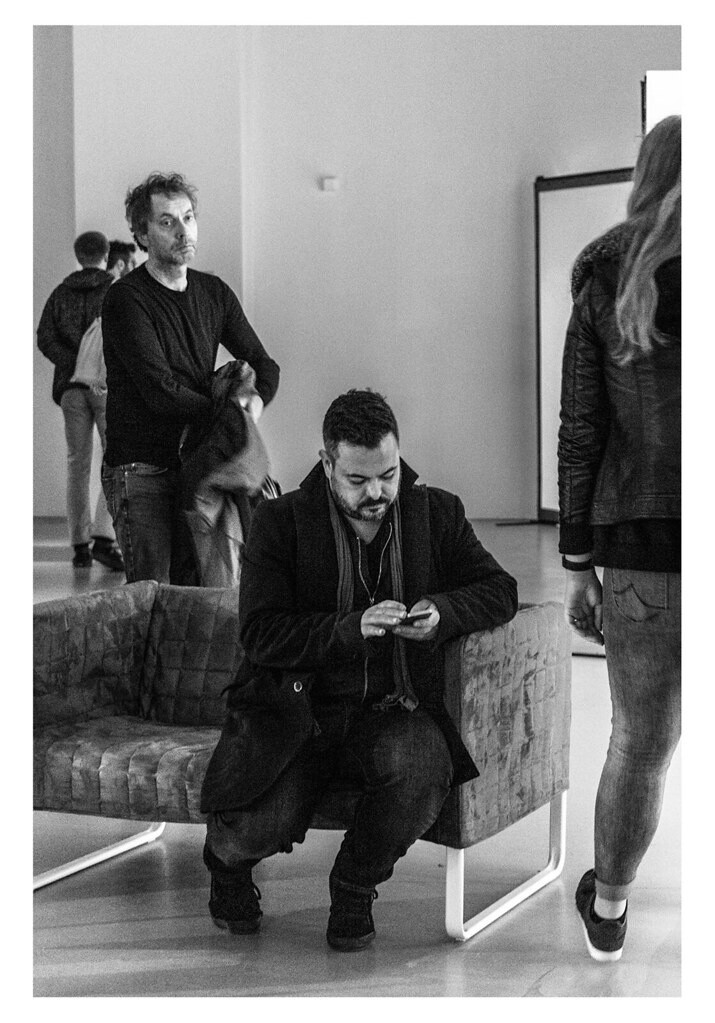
[37,231,127,570]
[199,386,520,951]
[107,238,137,279]
[100,172,281,587]
[553,117,682,963]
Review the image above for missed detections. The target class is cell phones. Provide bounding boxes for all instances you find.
[401,612,433,628]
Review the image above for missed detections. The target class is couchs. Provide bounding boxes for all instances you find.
[33,576,575,942]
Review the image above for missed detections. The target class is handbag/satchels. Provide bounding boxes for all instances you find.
[69,316,107,395]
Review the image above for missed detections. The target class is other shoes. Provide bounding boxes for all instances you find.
[89,544,124,572]
[73,554,92,568]
[327,896,377,948]
[203,871,266,932]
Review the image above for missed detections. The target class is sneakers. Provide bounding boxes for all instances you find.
[574,869,628,963]
